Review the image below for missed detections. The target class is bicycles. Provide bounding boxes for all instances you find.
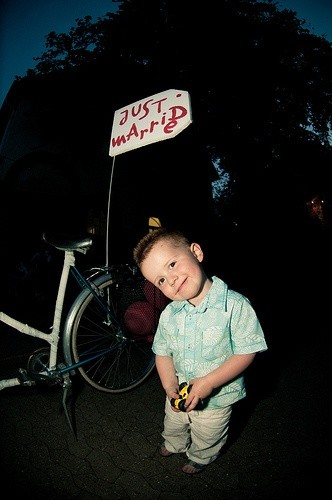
[1,230,169,441]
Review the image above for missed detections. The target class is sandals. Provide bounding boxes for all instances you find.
[158,442,174,457]
[182,459,208,474]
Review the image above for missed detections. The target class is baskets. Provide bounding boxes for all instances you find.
[111,267,169,338]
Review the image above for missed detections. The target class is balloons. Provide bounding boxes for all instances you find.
[125,278,168,336]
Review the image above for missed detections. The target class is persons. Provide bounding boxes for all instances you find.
[133,229,269,475]
